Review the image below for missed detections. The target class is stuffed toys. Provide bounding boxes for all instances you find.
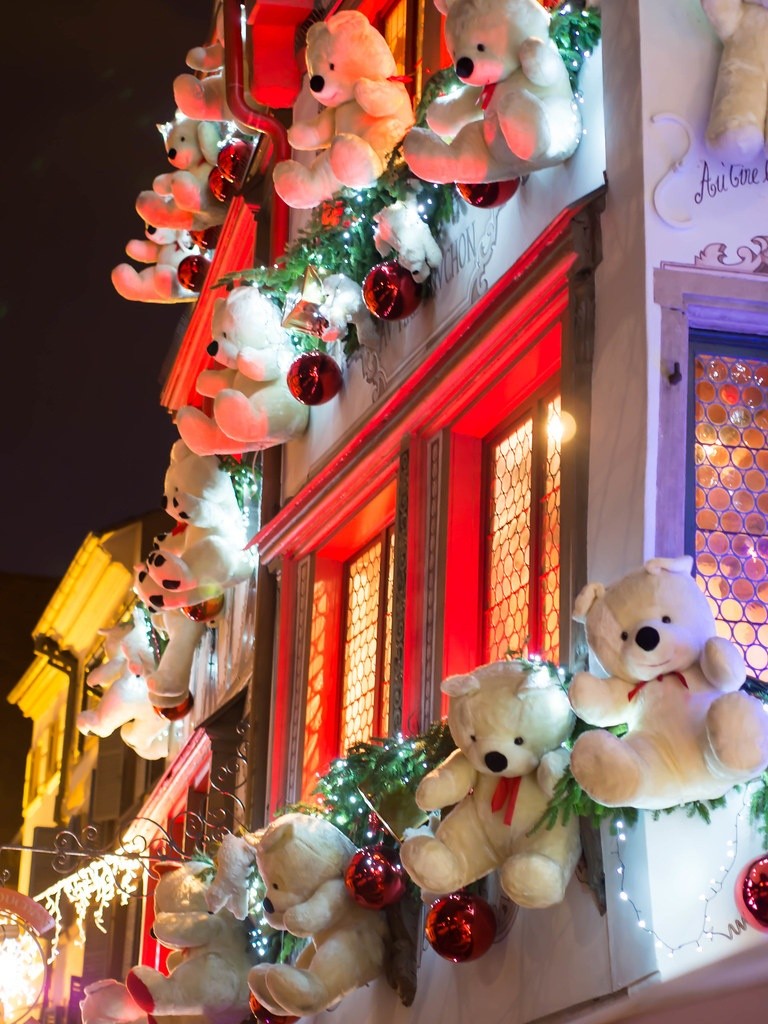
[176,286,310,456]
[76,439,254,760]
[402,0,582,184]
[272,10,415,209]
[318,273,381,350]
[127,859,264,1024]
[701,0,768,166]
[111,3,268,304]
[567,555,768,810]
[204,827,257,921]
[247,812,387,1016]
[399,659,582,909]
[78,978,148,1024]
[372,178,442,283]
